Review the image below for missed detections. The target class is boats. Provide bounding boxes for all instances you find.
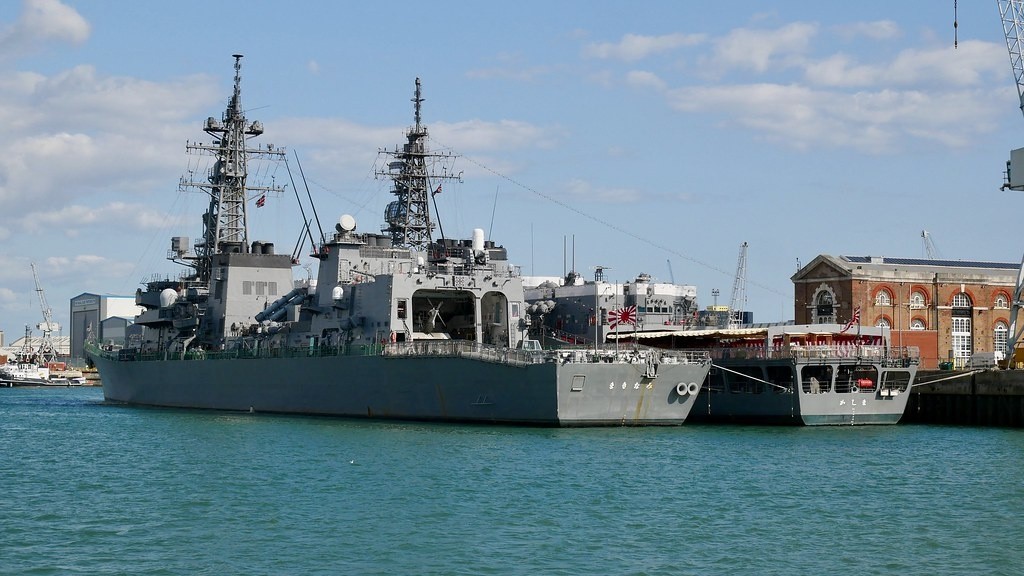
[0,361,89,388]
[79,45,717,429]
[510,263,919,428]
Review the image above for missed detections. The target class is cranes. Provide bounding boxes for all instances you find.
[28,261,63,366]
[726,241,749,325]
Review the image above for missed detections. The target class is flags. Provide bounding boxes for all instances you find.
[255,194,266,208]
[607,305,637,331]
[840,306,860,334]
